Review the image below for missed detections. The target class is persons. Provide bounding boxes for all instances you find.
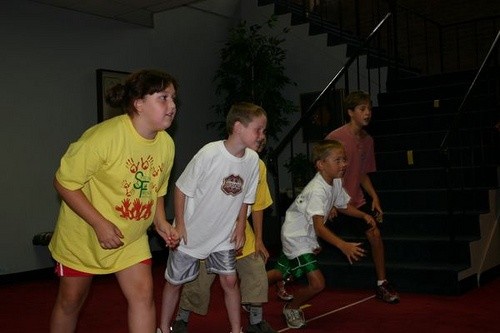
[277,91,400,302]
[243,139,376,329]
[48,69,179,333]
[160,102,267,333]
[171,136,275,333]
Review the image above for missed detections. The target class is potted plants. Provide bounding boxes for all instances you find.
[283,152,315,195]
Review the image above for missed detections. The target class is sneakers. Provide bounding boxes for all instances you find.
[377,281,400,303]
[276,280,293,301]
[282,304,306,329]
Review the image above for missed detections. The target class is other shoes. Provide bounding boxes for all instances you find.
[245,320,277,333]
[171,320,189,333]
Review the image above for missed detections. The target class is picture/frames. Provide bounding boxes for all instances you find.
[96,68,132,124]
